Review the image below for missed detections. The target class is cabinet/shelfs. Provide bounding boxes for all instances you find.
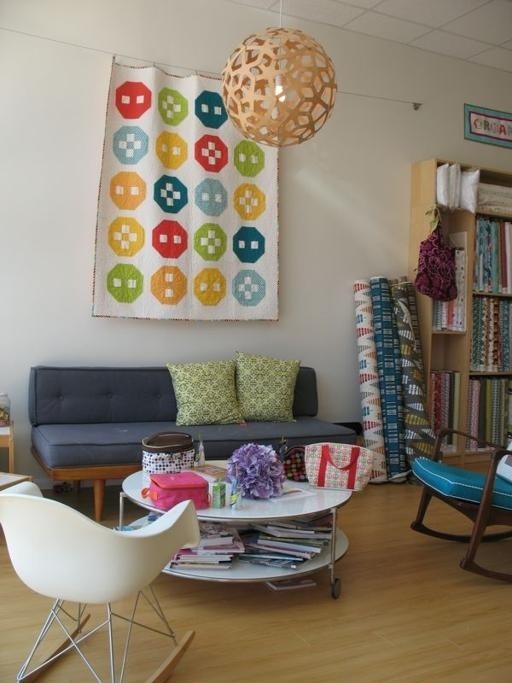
[406,154,512,464]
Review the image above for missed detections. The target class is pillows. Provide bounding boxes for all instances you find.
[164,350,300,427]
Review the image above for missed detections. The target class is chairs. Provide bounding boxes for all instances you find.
[0,471,32,492]
[0,481,200,682]
[0,393,15,474]
[411,428,512,587]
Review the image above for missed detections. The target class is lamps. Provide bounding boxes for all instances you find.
[221,0,338,147]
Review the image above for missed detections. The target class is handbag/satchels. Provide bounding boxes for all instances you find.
[414,217,458,301]
[303,442,374,491]
[140,472,210,511]
[281,445,308,481]
[140,431,195,480]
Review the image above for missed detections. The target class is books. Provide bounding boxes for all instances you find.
[265,575,318,591]
[184,461,227,483]
[142,510,332,571]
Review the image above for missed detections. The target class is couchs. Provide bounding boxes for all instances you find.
[28,365,357,525]
